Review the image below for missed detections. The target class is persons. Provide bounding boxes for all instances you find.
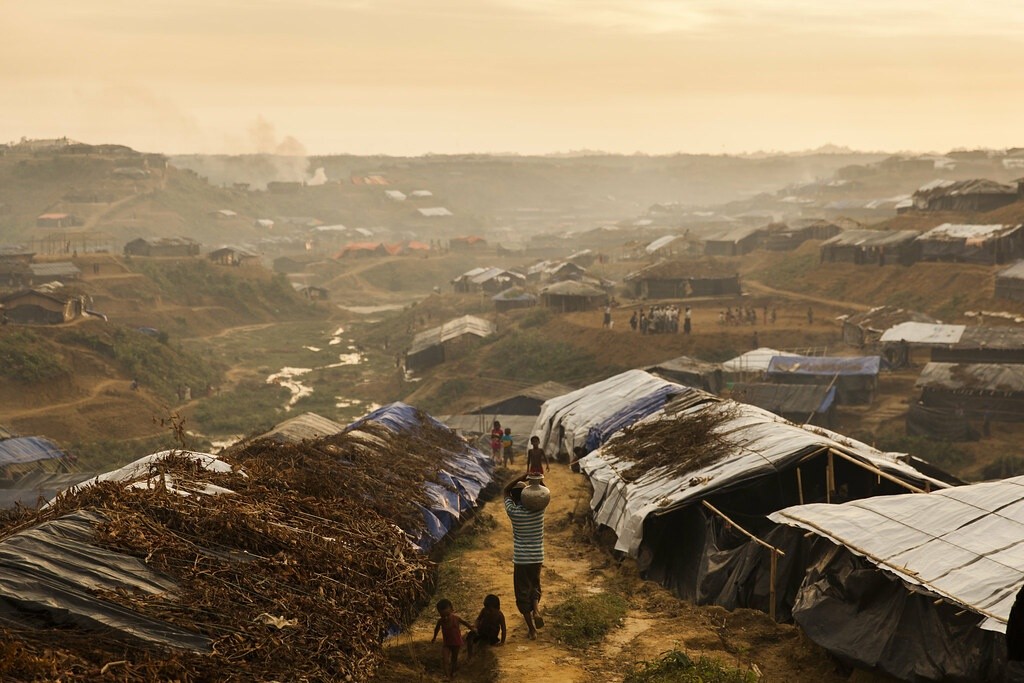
[526,436,550,485]
[124,376,223,402]
[464,594,508,665]
[599,300,813,351]
[491,420,504,464]
[500,428,514,468]
[430,598,477,682]
[503,472,548,640]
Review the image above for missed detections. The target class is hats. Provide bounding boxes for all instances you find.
[512,481,527,489]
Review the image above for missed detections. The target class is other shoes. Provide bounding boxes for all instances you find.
[536,616,545,629]
[527,630,537,640]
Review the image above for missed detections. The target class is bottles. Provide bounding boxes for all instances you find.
[521,473,550,511]
